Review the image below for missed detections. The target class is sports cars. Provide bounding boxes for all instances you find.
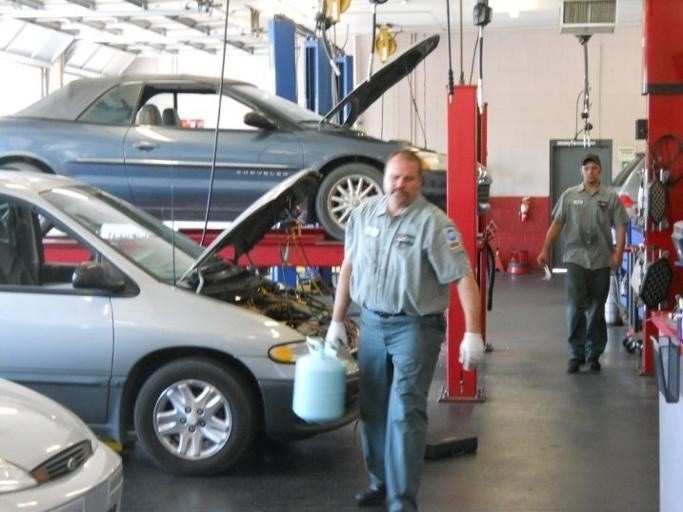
[0,32,495,240]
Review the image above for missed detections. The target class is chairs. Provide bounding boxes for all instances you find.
[0,219,35,285]
[134,104,183,127]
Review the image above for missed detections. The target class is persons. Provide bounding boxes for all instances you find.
[537,153,630,376]
[320,149,487,511]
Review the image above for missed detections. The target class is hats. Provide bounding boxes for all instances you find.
[581,153,599,165]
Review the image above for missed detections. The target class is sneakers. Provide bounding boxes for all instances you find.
[589,358,600,371]
[567,359,585,373]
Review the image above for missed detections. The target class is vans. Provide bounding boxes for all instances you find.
[606,151,646,218]
[0,169,361,481]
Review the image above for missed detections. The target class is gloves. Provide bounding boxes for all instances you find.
[325,321,350,353]
[458,332,485,370]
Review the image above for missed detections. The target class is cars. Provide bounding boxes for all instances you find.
[0,372,126,511]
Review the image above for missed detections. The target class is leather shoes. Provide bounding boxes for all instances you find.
[356,486,385,505]
